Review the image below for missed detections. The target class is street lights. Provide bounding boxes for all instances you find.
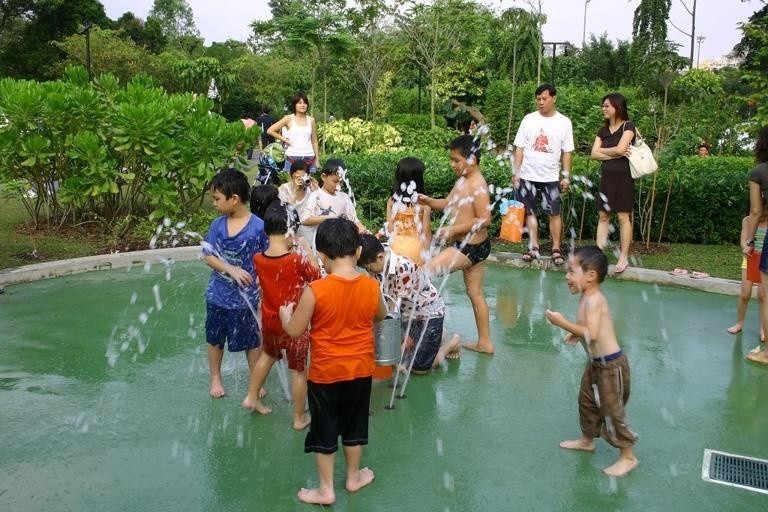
[540,41,568,86]
[408,59,422,115]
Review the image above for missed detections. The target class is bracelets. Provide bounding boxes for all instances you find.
[746,238,756,246]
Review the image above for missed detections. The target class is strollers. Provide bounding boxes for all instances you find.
[253,141,285,186]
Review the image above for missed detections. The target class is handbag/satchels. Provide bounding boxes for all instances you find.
[500,206,525,244]
[286,157,315,168]
[624,140,658,179]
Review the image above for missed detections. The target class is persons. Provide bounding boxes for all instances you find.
[328,111,336,123]
[266,94,320,174]
[696,146,709,158]
[728,192,768,342]
[238,108,275,159]
[200,134,494,504]
[512,85,574,266]
[591,93,636,273]
[545,247,639,476]
[742,125,768,364]
[460,121,476,136]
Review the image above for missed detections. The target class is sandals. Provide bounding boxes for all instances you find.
[523,247,540,261]
[614,258,629,272]
[551,248,565,264]
